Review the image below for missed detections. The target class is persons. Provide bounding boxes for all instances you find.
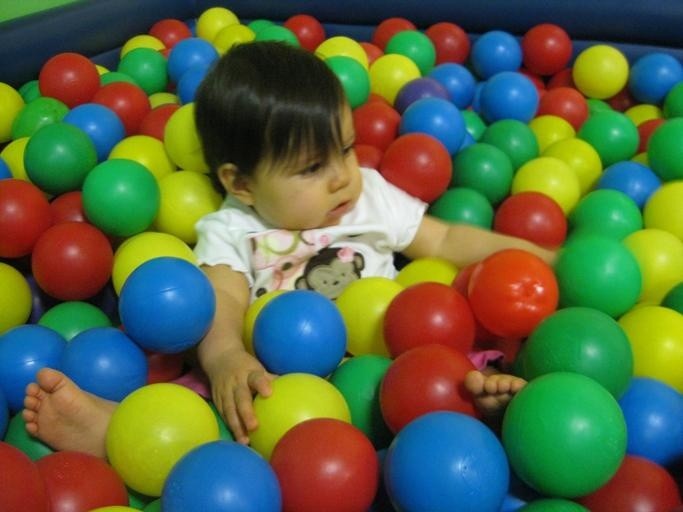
[24,39,556,458]
